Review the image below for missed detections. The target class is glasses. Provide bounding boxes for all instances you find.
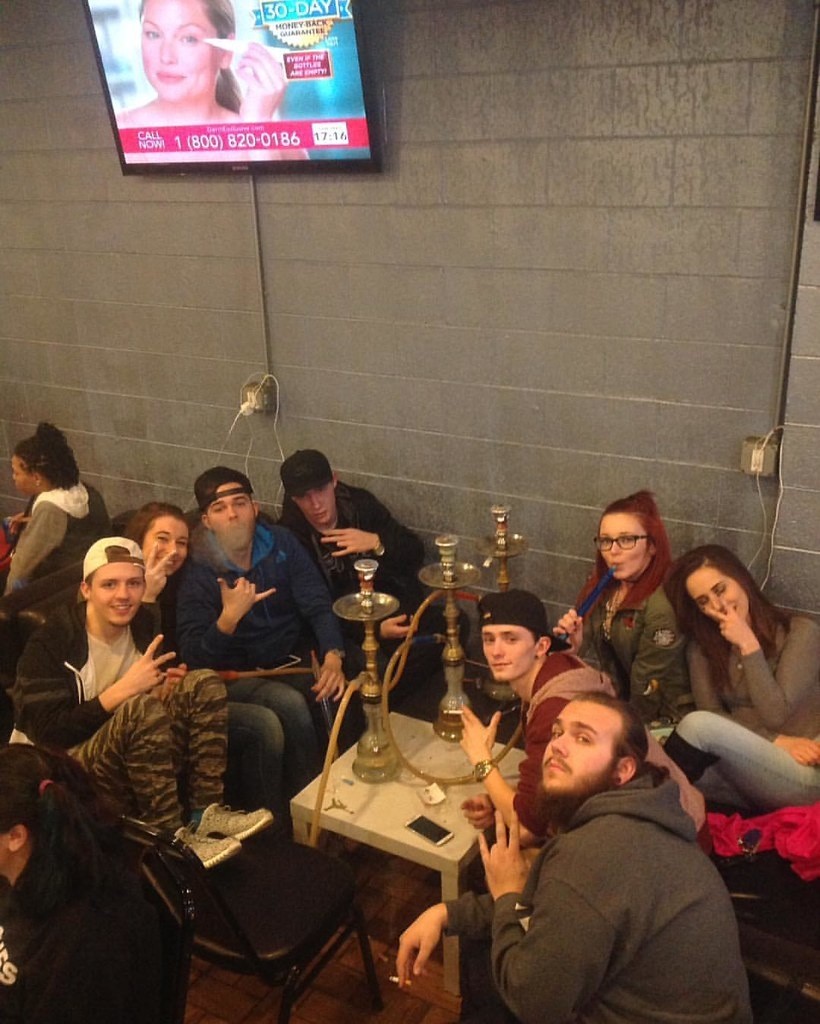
[594,534,651,551]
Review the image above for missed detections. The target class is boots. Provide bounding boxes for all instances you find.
[663,727,722,784]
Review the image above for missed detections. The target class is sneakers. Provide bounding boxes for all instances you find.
[194,802,274,841]
[177,823,242,868]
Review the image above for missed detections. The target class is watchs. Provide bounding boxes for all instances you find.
[475,760,499,783]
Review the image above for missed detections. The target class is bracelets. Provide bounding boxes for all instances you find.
[326,650,343,660]
[372,540,383,555]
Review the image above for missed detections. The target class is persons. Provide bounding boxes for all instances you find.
[0,611,38,689]
[183,467,273,536]
[662,544,819,815]
[172,466,367,760]
[460,589,618,848]
[13,537,274,869]
[116,0,311,164]
[396,689,754,1024]
[276,449,471,749]
[554,488,695,745]
[0,682,15,748]
[123,501,192,672]
[0,743,161,1023]
[0,420,111,622]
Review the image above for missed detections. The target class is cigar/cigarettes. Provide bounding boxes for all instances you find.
[390,976,411,985]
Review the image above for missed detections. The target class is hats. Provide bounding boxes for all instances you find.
[76,535,145,605]
[193,465,253,511]
[478,588,575,651]
[280,447,334,497]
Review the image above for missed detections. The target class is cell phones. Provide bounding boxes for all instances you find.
[404,814,454,846]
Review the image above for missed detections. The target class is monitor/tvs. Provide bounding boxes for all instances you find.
[82,0,382,175]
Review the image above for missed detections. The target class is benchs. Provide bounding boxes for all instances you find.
[698,724,820,1024]
[0,506,310,803]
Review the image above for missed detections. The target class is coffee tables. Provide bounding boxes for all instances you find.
[288,710,531,993]
[372,637,575,747]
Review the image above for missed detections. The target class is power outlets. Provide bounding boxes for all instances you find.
[240,383,277,416]
[740,434,784,475]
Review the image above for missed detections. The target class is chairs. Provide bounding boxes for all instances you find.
[121,805,386,1021]
